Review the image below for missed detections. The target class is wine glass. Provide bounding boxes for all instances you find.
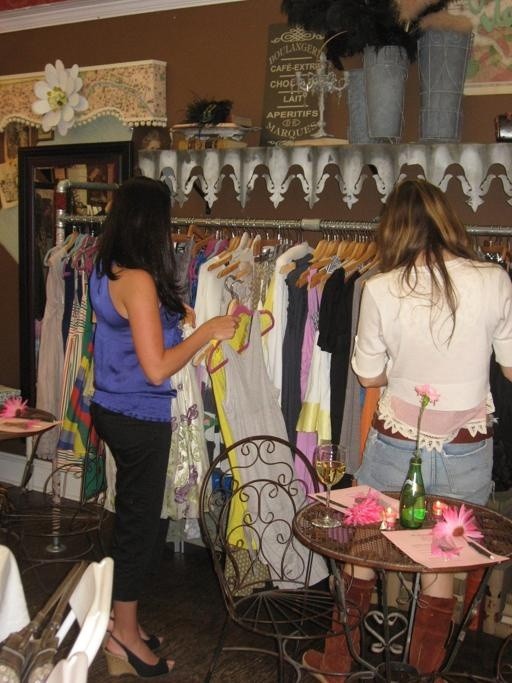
[310,446,347,529]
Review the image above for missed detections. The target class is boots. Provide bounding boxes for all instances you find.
[301,567,378,682]
[407,588,459,682]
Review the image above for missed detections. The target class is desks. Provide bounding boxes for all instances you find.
[0,400,58,550]
[293,490,510,682]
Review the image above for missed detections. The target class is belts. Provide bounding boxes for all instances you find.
[371,409,494,444]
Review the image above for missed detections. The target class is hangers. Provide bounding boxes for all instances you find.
[43,214,381,288]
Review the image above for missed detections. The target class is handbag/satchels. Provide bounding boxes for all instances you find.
[0,560,89,683]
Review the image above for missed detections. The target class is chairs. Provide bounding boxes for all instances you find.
[0,419,112,579]
[198,436,367,683]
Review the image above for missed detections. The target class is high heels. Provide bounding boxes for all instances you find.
[102,614,175,677]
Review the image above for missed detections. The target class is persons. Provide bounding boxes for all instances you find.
[301,177,512,683]
[88,176,241,677]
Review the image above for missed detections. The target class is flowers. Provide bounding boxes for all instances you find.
[411,384,442,522]
[0,395,30,420]
[429,503,485,559]
[32,60,90,136]
[316,487,387,528]
